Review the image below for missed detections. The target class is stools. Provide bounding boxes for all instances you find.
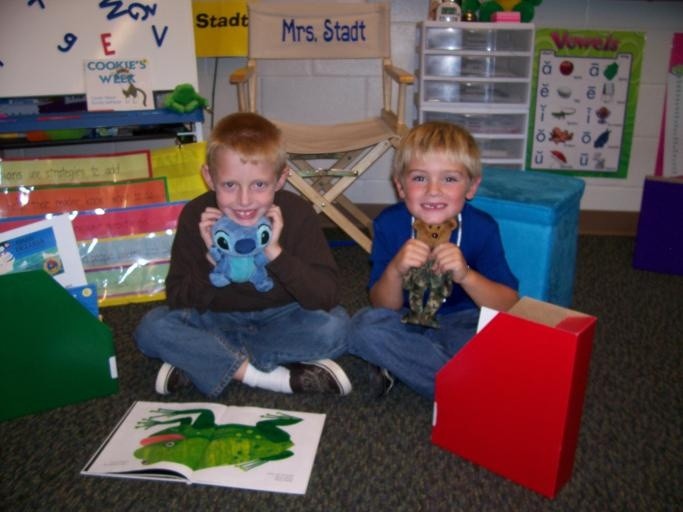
[467,167,584,307]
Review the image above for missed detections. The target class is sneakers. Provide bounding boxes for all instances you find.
[366,361,413,399]
[277,357,354,399]
[153,359,197,397]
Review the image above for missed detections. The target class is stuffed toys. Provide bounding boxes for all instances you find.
[208,217,275,295]
[399,220,460,330]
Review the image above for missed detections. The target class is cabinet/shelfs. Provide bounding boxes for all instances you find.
[414,22,534,170]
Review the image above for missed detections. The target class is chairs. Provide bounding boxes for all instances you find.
[228,1,415,251]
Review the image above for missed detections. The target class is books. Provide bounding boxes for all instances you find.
[80,398,327,495]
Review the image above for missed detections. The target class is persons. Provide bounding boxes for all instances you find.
[347,120,522,402]
[135,112,354,400]
